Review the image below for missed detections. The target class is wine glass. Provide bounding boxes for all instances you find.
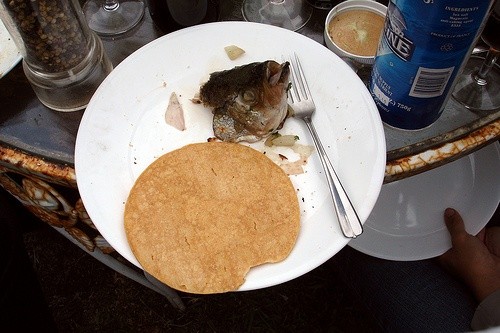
[453,0,500,112]
[241,0,313,31]
[82,0,145,36]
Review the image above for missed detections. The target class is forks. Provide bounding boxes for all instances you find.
[276,51,364,240]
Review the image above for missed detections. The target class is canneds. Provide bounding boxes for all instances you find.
[367,0,494,132]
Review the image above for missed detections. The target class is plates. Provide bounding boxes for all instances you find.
[347,103,500,263]
[74,21,387,292]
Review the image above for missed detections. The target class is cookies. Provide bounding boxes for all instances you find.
[123,141,301,295]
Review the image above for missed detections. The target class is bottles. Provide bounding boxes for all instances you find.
[147,0,220,32]
[366,0,494,132]
[0,0,113,112]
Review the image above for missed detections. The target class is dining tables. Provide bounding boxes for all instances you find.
[0,0,500,333]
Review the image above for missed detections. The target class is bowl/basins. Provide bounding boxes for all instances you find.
[324,0,388,63]
